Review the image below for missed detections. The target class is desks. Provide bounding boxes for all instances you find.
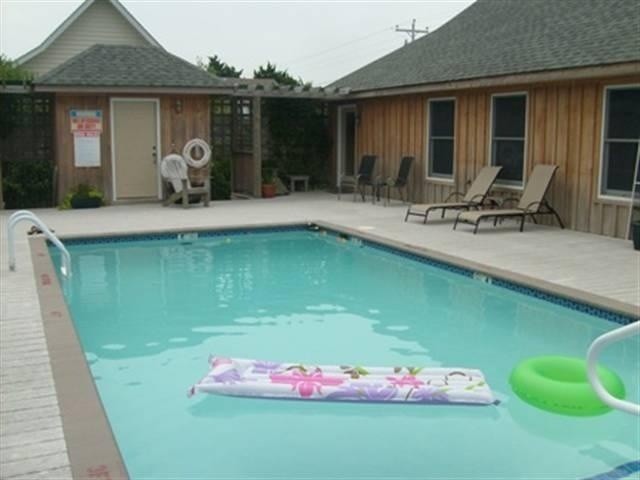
[163,175,215,208]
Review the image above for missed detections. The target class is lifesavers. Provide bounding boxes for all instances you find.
[510,354,626,415]
[161,154,187,182]
[182,138,211,169]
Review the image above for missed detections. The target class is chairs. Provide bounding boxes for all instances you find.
[279,163,310,192]
[404,164,565,235]
[336,154,414,206]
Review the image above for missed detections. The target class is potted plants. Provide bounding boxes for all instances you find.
[60,182,107,209]
